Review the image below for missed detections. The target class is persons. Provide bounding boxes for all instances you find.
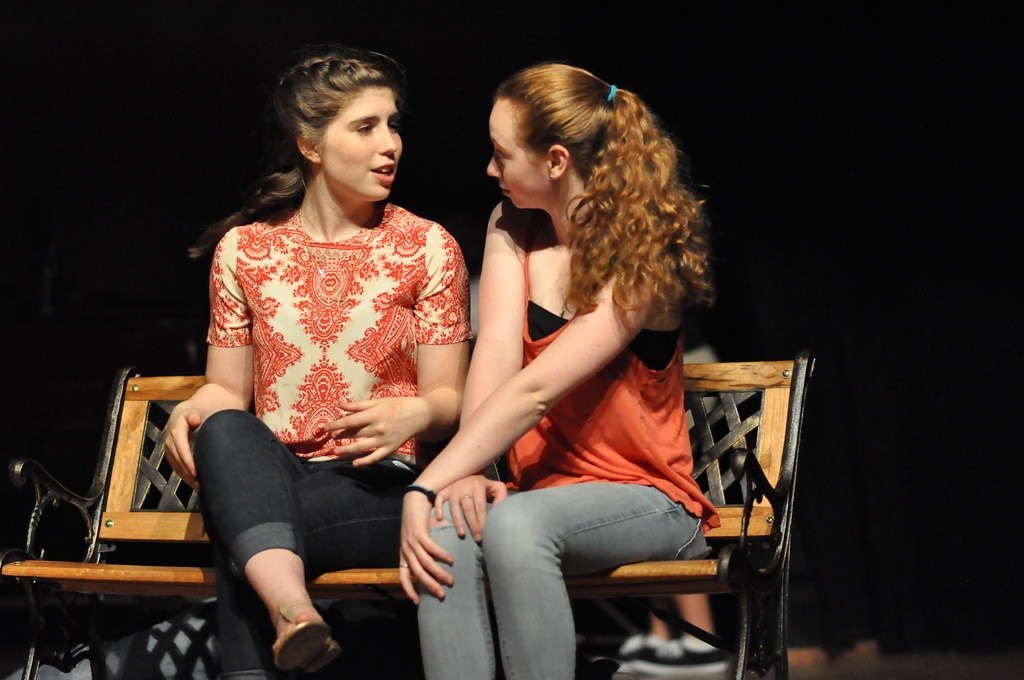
[617,584,730,676]
[164,42,474,680]
[398,63,722,680]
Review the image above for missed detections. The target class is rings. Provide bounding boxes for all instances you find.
[462,494,474,500]
[398,563,410,568]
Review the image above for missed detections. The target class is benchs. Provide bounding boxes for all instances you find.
[0,352,816,680]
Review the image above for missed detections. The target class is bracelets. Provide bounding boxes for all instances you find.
[403,483,438,506]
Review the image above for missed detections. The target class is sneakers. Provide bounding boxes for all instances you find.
[592,631,729,677]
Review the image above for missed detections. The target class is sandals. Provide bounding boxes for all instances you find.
[271,599,342,672]
